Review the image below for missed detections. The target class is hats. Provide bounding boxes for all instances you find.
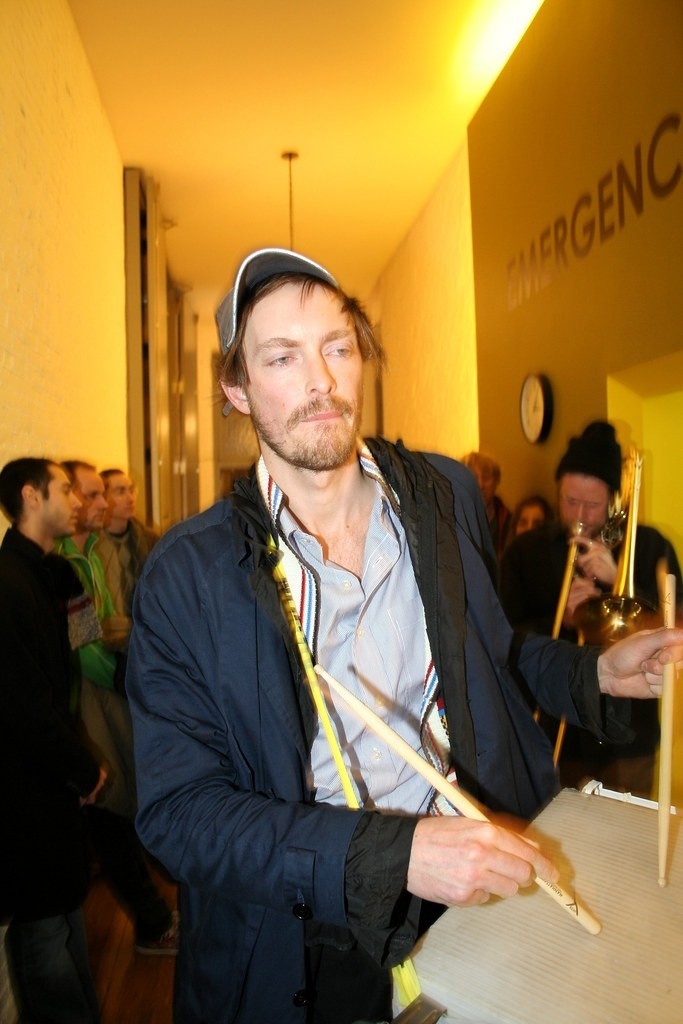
[554,420,623,492]
[214,248,341,418]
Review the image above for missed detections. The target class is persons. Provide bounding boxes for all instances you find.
[0,457,181,1024]
[123,248,683,1024]
[459,421,683,799]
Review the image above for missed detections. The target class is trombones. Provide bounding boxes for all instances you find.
[525,444,659,770]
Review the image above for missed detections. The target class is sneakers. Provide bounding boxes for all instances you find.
[134,919,187,954]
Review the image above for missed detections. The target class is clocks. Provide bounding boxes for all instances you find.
[519,372,555,444]
